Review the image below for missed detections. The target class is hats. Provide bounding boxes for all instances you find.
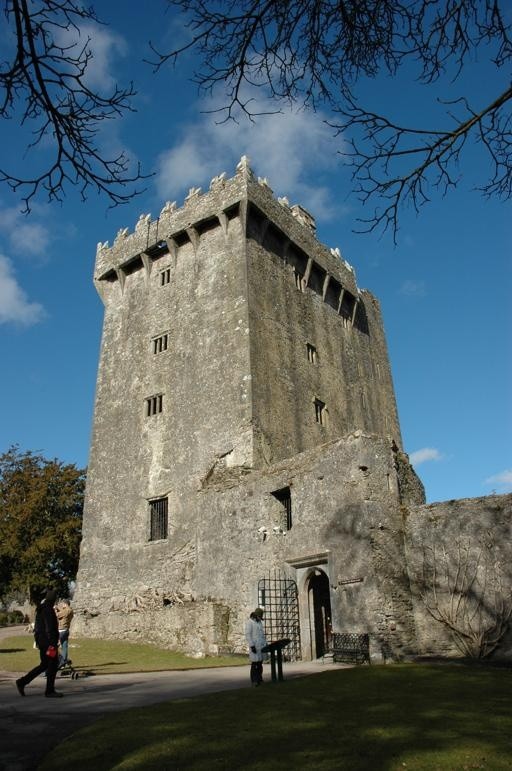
[254,608,263,616]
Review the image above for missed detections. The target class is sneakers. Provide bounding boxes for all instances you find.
[16,679,25,697]
[45,691,63,698]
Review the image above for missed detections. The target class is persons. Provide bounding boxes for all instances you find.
[245,608,268,689]
[52,599,75,672]
[15,592,65,698]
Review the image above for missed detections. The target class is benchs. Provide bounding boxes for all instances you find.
[322,633,371,666]
